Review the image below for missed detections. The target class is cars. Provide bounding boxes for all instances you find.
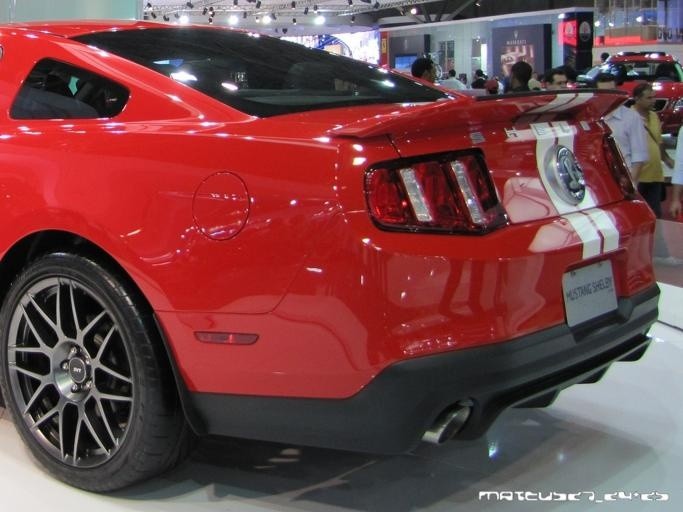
[1,21,660,494]
[600,51,683,137]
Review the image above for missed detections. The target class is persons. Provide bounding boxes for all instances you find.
[412,53,683,267]
